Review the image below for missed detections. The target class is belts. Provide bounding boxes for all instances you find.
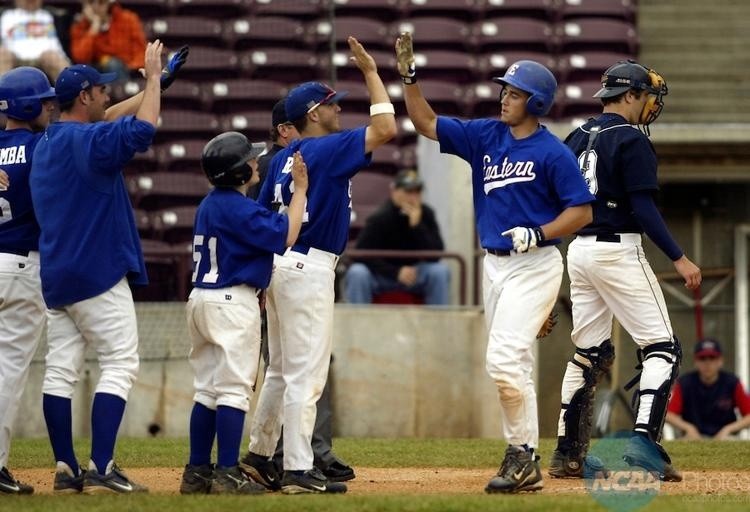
[596,235,621,245]
[487,246,539,258]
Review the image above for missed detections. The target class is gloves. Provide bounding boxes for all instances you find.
[393,30,420,86]
[160,47,188,90]
[499,225,544,254]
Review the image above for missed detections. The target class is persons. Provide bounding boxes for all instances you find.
[548,58,702,481]
[341,169,451,304]
[2,3,395,497]
[662,337,750,441]
[396,32,597,494]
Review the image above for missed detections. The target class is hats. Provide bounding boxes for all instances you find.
[591,63,651,98]
[272,82,349,124]
[202,131,268,188]
[693,339,727,360]
[391,170,426,193]
[50,64,122,94]
[492,59,557,116]
[0,65,61,120]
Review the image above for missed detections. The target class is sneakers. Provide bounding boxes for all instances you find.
[178,442,358,493]
[483,449,544,494]
[622,439,683,483]
[54,466,87,494]
[548,452,611,480]
[79,469,149,497]
[0,465,35,493]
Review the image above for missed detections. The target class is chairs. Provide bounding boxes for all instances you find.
[0,0,651,309]
